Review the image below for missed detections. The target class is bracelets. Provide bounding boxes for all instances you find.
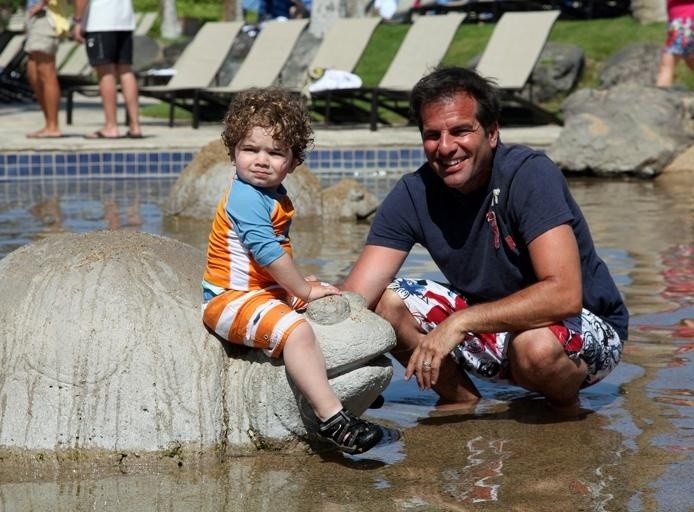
[73,17,81,22]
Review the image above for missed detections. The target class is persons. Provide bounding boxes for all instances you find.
[335,68,630,420]
[199,86,383,453]
[654,1,693,87]
[255,0,309,23]
[18,0,72,139]
[72,0,144,140]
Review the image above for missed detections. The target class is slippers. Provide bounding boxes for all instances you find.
[83,130,143,138]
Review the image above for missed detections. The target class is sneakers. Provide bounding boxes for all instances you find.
[314,395,384,454]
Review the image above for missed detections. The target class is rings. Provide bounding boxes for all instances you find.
[422,359,432,366]
